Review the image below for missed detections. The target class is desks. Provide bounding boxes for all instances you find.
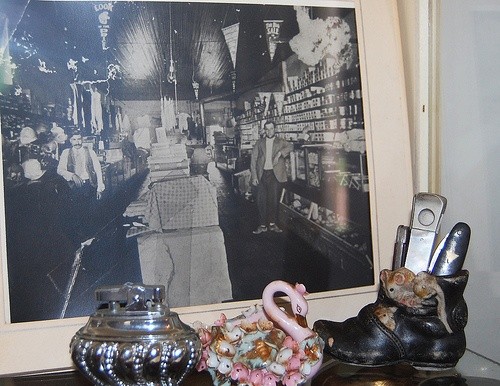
[1,346,500,385]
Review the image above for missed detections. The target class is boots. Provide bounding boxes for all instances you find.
[313,270,470,371]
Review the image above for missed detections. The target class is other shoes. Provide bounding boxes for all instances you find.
[252,227,268,233]
[270,225,283,233]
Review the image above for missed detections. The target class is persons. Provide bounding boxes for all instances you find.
[56,131,105,246]
[121,134,143,169]
[249,121,292,235]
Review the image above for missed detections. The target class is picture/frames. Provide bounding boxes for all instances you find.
[1,1,414,377]
